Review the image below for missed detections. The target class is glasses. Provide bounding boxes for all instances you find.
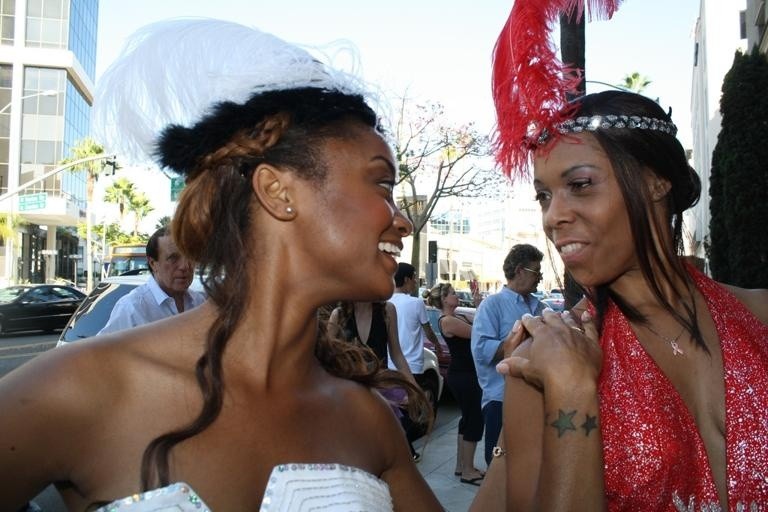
[522,266,541,278]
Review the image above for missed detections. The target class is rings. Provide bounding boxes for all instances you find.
[568,324,586,334]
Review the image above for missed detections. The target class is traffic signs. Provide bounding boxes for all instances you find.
[170,178,188,201]
[18,192,48,210]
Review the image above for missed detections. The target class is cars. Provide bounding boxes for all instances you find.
[0,282,87,336]
[57,267,226,347]
[411,288,586,443]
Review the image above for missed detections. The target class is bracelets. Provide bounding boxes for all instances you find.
[489,443,508,459]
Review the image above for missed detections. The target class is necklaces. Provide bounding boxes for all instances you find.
[631,278,700,359]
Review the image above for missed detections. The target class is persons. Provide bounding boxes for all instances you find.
[327,297,426,413]
[423,281,489,487]
[469,243,553,467]
[388,262,444,463]
[88,224,206,338]
[495,89,767,510]
[0,18,563,511]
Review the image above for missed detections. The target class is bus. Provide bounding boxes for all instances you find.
[105,244,225,276]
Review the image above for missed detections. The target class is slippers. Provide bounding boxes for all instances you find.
[454,468,478,475]
[460,475,483,487]
